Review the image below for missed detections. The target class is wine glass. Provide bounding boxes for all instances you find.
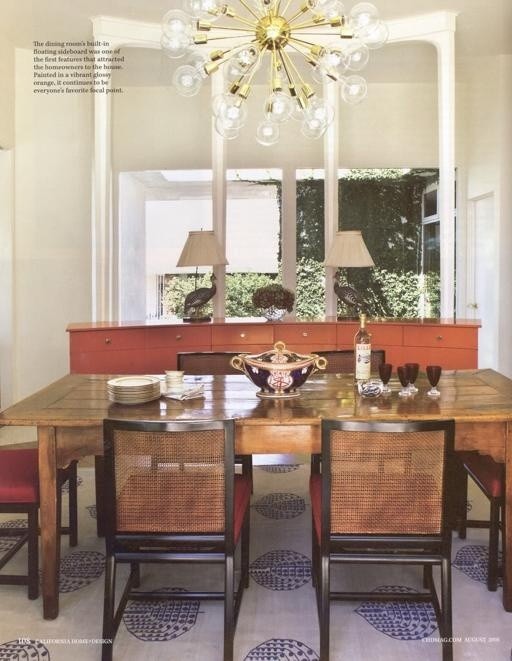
[404,361,419,393]
[426,365,441,397]
[397,366,412,398]
[378,362,393,394]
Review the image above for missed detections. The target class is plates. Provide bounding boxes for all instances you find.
[105,375,162,405]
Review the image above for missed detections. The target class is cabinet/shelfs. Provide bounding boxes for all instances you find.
[211,326,274,354]
[337,326,404,372]
[275,325,338,355]
[405,326,477,372]
[146,328,211,374]
[67,330,145,374]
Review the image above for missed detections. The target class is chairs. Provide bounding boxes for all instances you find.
[461,454,505,592]
[0,448,79,599]
[176,351,253,377]
[311,349,385,376]
[310,416,455,661]
[101,418,254,661]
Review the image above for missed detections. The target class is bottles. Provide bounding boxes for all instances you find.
[351,313,372,387]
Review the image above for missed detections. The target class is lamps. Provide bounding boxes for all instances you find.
[320,229,375,321]
[175,227,230,323]
[158,0,391,148]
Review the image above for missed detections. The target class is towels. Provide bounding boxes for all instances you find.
[163,385,207,402]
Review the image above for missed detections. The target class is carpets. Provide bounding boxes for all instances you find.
[0,461,512,661]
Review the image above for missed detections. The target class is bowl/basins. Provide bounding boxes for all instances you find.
[164,370,185,388]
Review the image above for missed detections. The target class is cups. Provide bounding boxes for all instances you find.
[356,377,384,399]
